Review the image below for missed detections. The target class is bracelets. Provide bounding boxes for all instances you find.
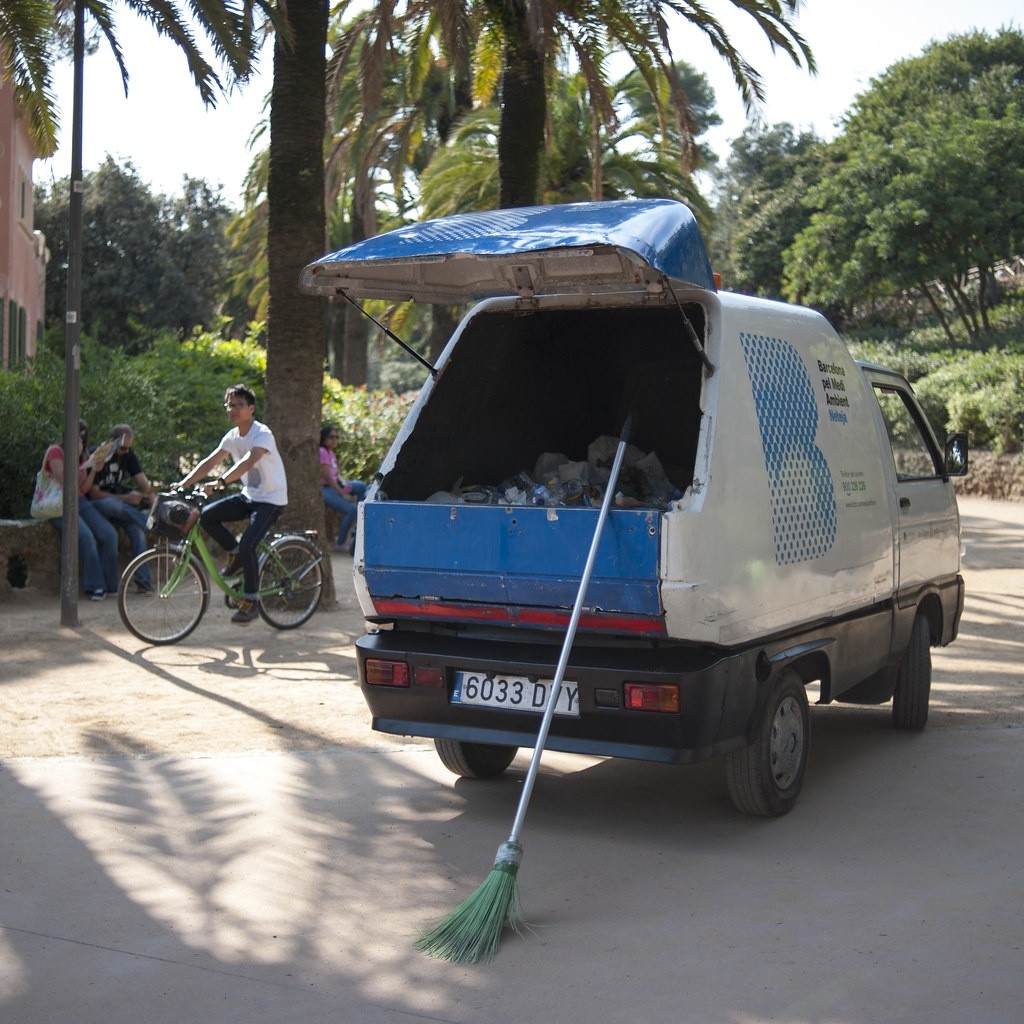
[218,477,226,487]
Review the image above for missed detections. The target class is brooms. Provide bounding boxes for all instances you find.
[413,392,641,970]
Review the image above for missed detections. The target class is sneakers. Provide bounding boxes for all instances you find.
[218,552,244,578]
[231,600,259,622]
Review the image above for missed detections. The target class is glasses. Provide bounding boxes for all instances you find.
[330,435,339,439]
[224,403,244,410]
[120,446,132,451]
[80,434,86,439]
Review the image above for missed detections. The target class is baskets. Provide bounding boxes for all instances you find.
[145,493,201,539]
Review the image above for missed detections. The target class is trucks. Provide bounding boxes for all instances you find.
[296,197,972,822]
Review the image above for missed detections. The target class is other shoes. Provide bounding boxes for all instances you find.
[91,588,119,600]
[334,543,350,552]
[137,586,155,596]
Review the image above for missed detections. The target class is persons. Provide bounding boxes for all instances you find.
[43,420,120,601]
[84,422,160,596]
[320,426,366,552]
[169,382,288,624]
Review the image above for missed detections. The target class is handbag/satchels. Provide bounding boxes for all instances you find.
[30,444,64,519]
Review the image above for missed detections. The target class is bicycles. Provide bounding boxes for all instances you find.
[118,488,327,647]
